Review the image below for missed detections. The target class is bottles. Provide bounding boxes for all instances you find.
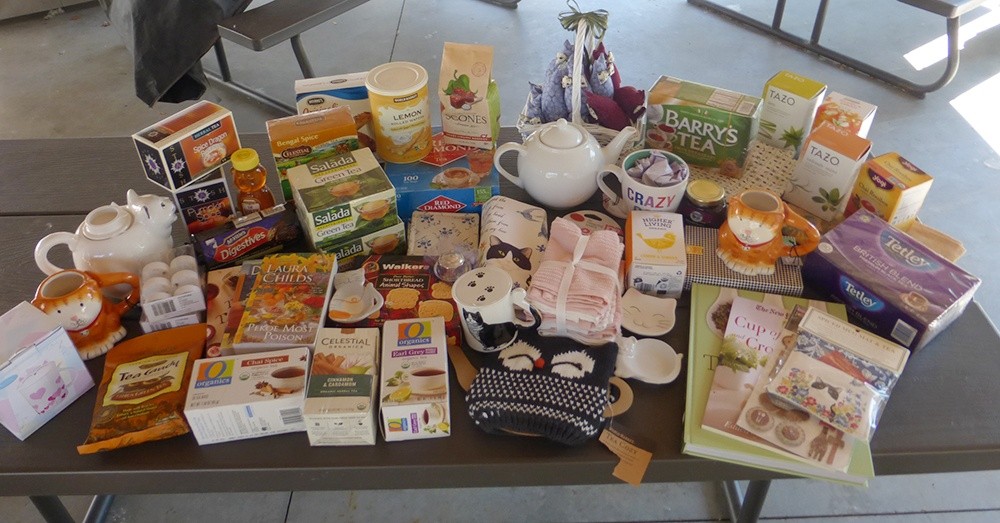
[231,148,276,216]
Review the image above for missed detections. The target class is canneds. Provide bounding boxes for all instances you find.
[678,178,726,227]
[365,61,433,164]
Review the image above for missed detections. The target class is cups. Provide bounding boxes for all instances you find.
[596,148,692,213]
[403,368,445,391]
[34,270,140,358]
[327,181,400,255]
[269,365,305,392]
[645,122,678,149]
[421,408,440,428]
[19,362,76,417]
[717,190,820,276]
[451,267,541,351]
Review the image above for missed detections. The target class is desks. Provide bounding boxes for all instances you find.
[0,123,1000,523]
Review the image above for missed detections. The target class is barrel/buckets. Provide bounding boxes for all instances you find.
[364,62,430,163]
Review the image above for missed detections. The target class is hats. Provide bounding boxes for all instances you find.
[466,326,617,447]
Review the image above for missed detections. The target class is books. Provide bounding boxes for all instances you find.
[681,283,911,489]
[205,253,338,357]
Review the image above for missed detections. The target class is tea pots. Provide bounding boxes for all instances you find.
[493,119,637,208]
[34,188,178,302]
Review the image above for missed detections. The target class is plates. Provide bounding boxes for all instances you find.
[410,389,447,396]
[432,402,445,426]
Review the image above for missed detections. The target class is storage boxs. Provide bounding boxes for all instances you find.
[132,100,240,192]
[183,347,310,446]
[301,326,379,448]
[320,216,408,271]
[783,120,873,220]
[137,310,200,333]
[135,243,206,321]
[294,72,373,147]
[813,92,878,142]
[758,71,827,155]
[685,225,805,298]
[646,76,765,179]
[0,297,95,442]
[173,179,282,270]
[285,145,398,250]
[267,108,361,200]
[844,150,933,226]
[378,317,452,443]
[479,196,549,289]
[802,207,981,355]
[623,208,688,299]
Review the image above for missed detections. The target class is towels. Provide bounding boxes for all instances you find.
[524,216,626,348]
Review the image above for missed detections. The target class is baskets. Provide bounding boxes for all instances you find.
[517,15,645,163]
